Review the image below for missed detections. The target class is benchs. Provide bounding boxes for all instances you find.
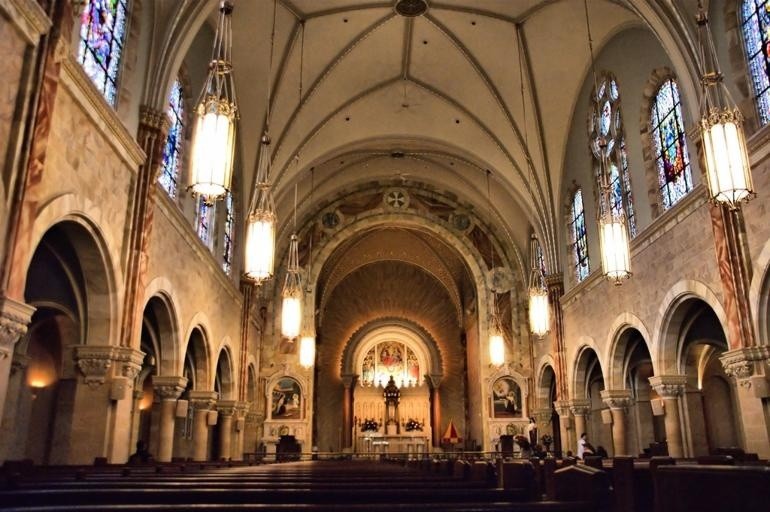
[2,457,768,512]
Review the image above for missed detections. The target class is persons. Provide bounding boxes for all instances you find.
[535,445,547,459]
[505,391,518,413]
[129,440,149,465]
[562,433,609,466]
[529,418,537,445]
[274,395,285,416]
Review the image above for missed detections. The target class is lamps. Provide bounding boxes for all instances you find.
[185,0,239,210]
[687,4,755,213]
[513,22,551,336]
[582,5,633,291]
[275,14,304,343]
[484,168,508,365]
[240,0,276,292]
[298,331,317,374]
